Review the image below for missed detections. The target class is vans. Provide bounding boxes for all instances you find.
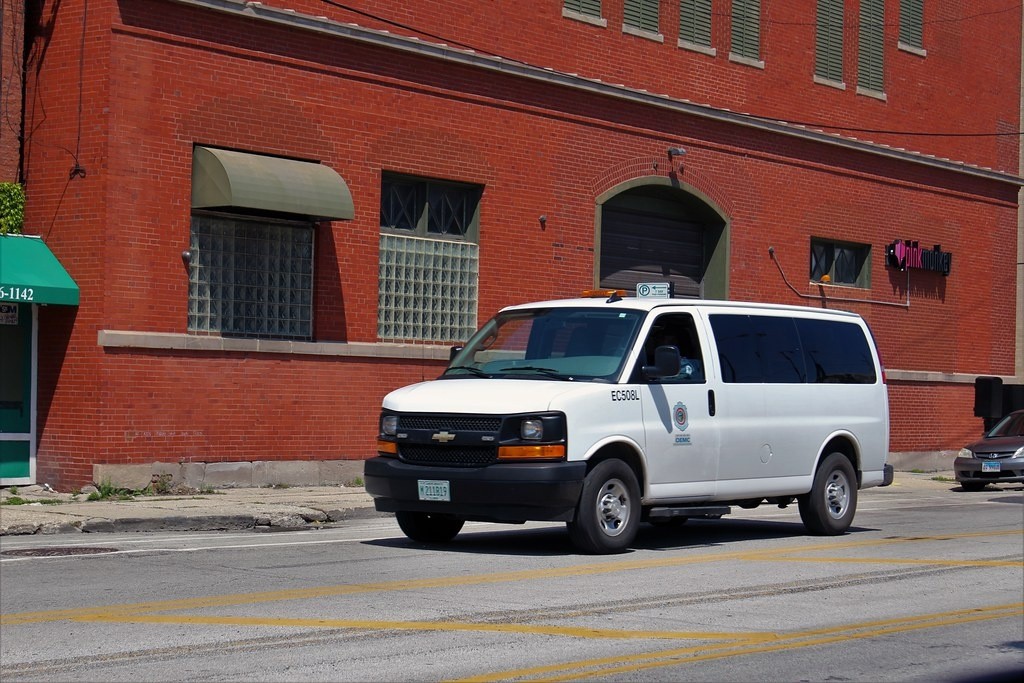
[363,287,896,556]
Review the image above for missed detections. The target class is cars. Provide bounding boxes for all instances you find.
[953,409,1024,493]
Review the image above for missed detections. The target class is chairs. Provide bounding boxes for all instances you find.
[565,327,602,356]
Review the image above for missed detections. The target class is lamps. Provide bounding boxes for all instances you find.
[667,147,686,156]
